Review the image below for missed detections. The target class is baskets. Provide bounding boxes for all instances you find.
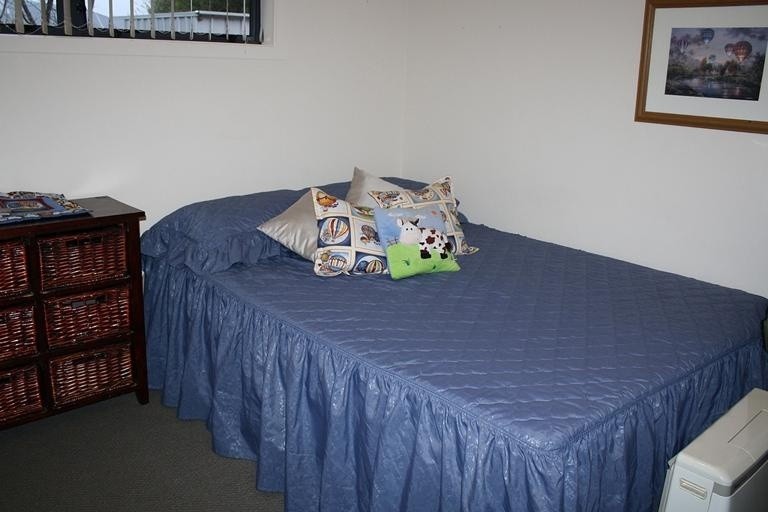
[39,343,135,406]
[34,227,128,290]
[40,283,131,350]
[0,240,43,425]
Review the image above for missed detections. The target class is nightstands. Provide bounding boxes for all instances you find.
[0,190,150,427]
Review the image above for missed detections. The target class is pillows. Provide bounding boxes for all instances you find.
[134,186,301,280]
[368,176,481,259]
[368,204,461,280]
[308,185,389,281]
[341,166,404,212]
[258,187,319,261]
[311,175,467,221]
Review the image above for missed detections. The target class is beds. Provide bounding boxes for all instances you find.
[141,174,767,512]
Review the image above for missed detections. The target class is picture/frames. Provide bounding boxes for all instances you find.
[632,0,767,133]
